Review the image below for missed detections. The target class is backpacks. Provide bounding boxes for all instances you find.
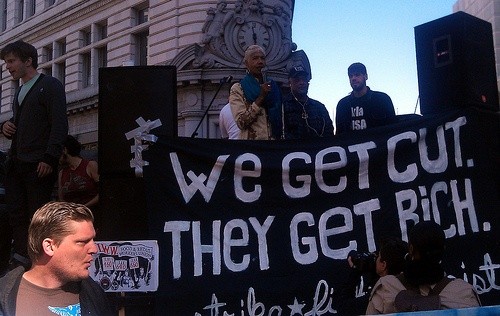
[395,273,455,313]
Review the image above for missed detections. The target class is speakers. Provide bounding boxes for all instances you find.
[414,11,499,116]
[98,64,178,176]
[95,174,146,241]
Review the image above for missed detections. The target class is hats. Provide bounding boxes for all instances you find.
[409,221,446,252]
[287,65,309,77]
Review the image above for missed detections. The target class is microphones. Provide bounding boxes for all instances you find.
[261,67,267,84]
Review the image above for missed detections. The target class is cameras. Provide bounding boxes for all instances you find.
[348,249,372,272]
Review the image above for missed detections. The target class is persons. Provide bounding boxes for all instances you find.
[0,39,68,263]
[336,63,398,136]
[57,134,100,241]
[337,220,483,316]
[282,64,334,140]
[0,202,120,316]
[218,44,285,140]
